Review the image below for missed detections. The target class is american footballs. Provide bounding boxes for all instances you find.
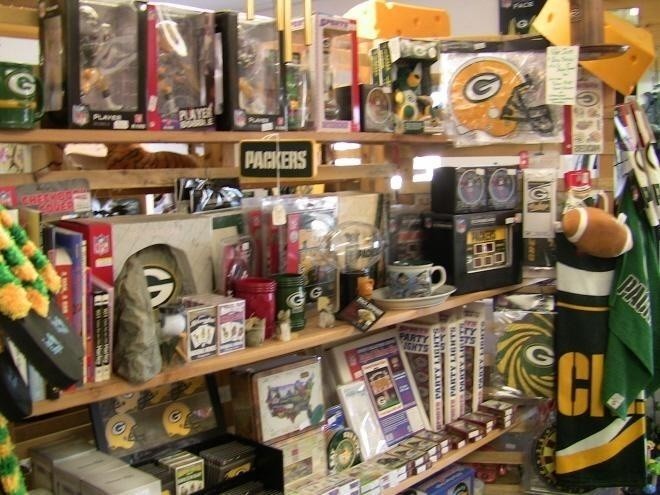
[563,207,634,258]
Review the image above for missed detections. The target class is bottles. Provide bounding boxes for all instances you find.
[0,36,41,129]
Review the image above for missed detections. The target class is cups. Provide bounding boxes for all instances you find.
[271,273,306,330]
[385,259,447,300]
[234,278,277,339]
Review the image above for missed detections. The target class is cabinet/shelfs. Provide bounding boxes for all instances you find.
[0,0,618,495]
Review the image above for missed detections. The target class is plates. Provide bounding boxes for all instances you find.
[371,285,458,311]
